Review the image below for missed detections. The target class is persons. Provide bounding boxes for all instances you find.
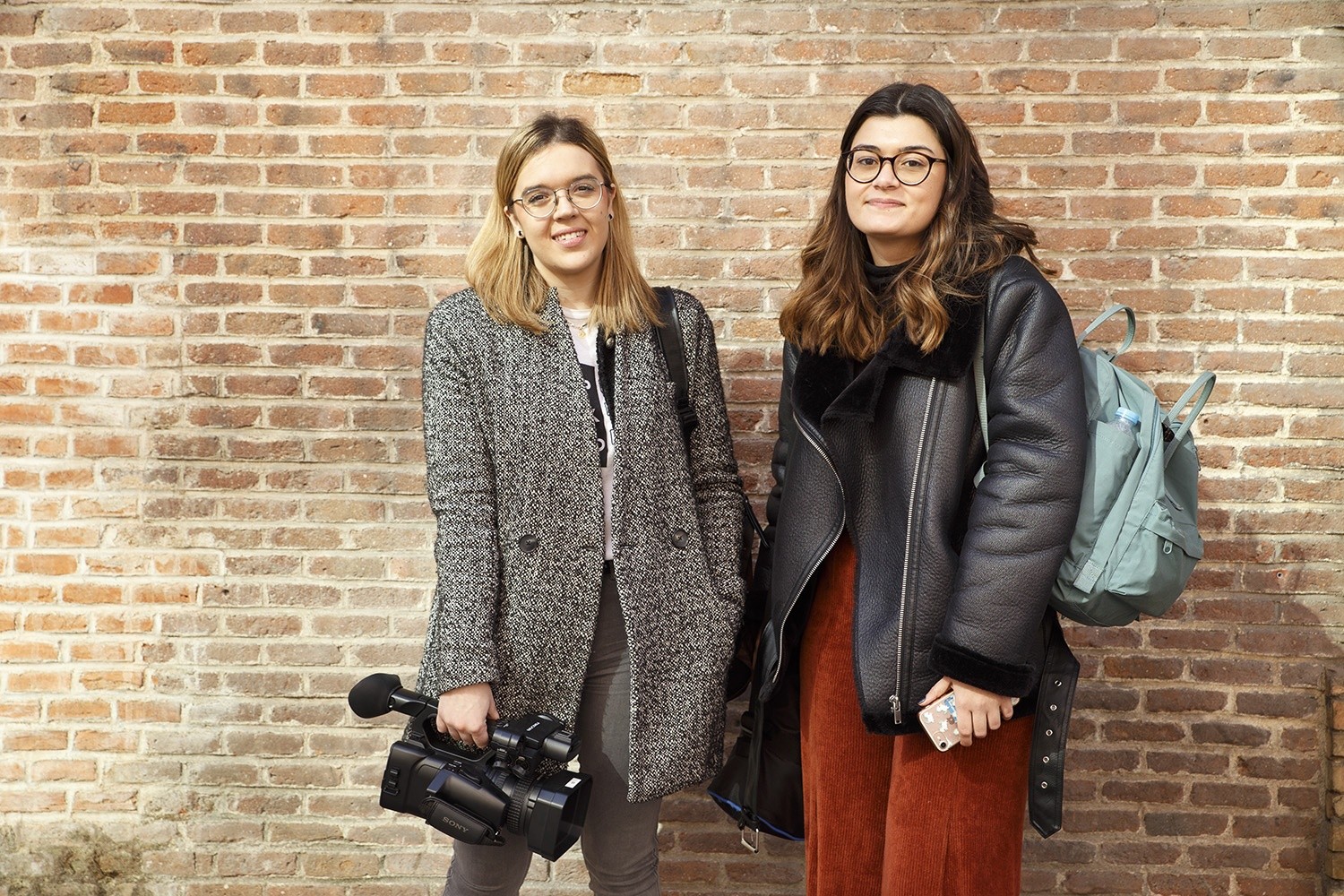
[402,112,759,896]
[748,84,1092,895]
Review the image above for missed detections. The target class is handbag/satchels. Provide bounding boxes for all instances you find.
[705,640,804,853]
[647,283,774,708]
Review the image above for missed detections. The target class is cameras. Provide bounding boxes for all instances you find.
[349,672,593,862]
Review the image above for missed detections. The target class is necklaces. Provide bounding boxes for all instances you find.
[559,310,597,337]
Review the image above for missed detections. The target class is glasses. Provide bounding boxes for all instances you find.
[840,148,948,185]
[509,178,609,218]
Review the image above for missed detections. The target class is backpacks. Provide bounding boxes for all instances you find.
[972,251,1219,628]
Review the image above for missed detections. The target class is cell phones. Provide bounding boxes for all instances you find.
[915,690,1021,753]
